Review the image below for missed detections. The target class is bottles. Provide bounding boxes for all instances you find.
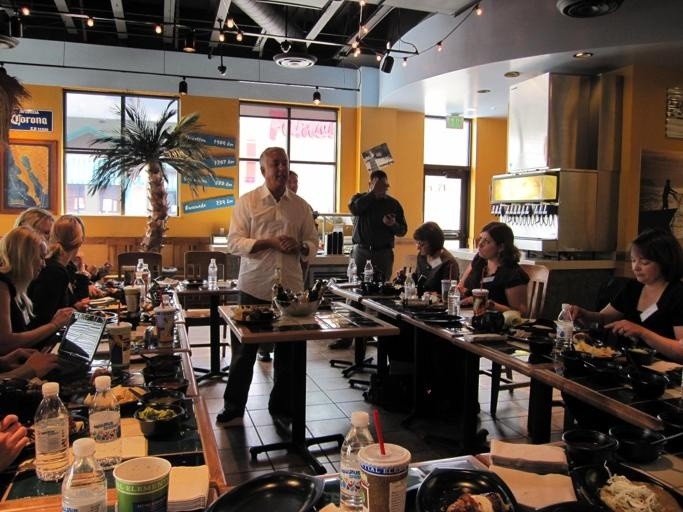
[555,304,573,352]
[337,410,374,504]
[272,266,335,303]
[32,381,71,483]
[403,273,416,297]
[87,374,122,470]
[447,279,461,316]
[87,258,179,346]
[346,258,357,283]
[207,258,217,288]
[363,259,372,282]
[58,437,108,511]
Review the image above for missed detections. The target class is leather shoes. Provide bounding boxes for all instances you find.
[327,339,351,348]
[216,407,245,423]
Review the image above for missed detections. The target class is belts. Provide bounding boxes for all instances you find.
[356,244,393,250]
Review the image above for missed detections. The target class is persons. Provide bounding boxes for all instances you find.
[256,172,313,361]
[327,171,407,350]
[216,147,318,423]
[562,228,683,452]
[0,207,104,473]
[412,222,459,297]
[663,180,678,210]
[457,222,530,354]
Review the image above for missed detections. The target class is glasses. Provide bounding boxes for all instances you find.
[415,240,425,247]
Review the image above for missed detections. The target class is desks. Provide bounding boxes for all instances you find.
[328,281,680,448]
[1,280,225,512]
[175,278,241,385]
[311,446,682,509]
[218,301,400,475]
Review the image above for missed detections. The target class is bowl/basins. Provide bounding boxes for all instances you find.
[620,343,668,399]
[559,351,595,366]
[133,354,191,441]
[526,336,553,353]
[271,291,324,318]
[550,418,666,462]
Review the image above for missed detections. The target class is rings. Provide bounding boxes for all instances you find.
[618,328,626,333]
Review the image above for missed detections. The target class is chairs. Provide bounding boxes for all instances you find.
[118,252,162,324]
[184,252,227,357]
[479,264,549,417]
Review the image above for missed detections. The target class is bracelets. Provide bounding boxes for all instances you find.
[487,299,495,309]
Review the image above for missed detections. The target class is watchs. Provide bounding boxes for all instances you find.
[300,242,308,255]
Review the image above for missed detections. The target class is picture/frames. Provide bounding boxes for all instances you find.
[0,138,58,215]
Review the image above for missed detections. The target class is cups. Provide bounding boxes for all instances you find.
[357,442,411,511]
[104,321,133,367]
[441,280,452,303]
[112,455,171,511]
[471,288,488,315]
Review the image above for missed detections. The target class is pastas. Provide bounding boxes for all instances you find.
[601,461,660,512]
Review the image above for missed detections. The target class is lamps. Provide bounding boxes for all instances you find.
[179,77,188,98]
[183,29,196,54]
[381,50,394,74]
[313,85,321,105]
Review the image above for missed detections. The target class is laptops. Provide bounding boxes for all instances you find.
[48,311,106,378]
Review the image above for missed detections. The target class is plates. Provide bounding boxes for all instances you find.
[230,303,279,324]
[398,303,466,324]
[567,463,683,512]
[205,470,325,512]
[414,465,517,512]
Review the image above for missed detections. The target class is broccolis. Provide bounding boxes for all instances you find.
[138,407,178,419]
